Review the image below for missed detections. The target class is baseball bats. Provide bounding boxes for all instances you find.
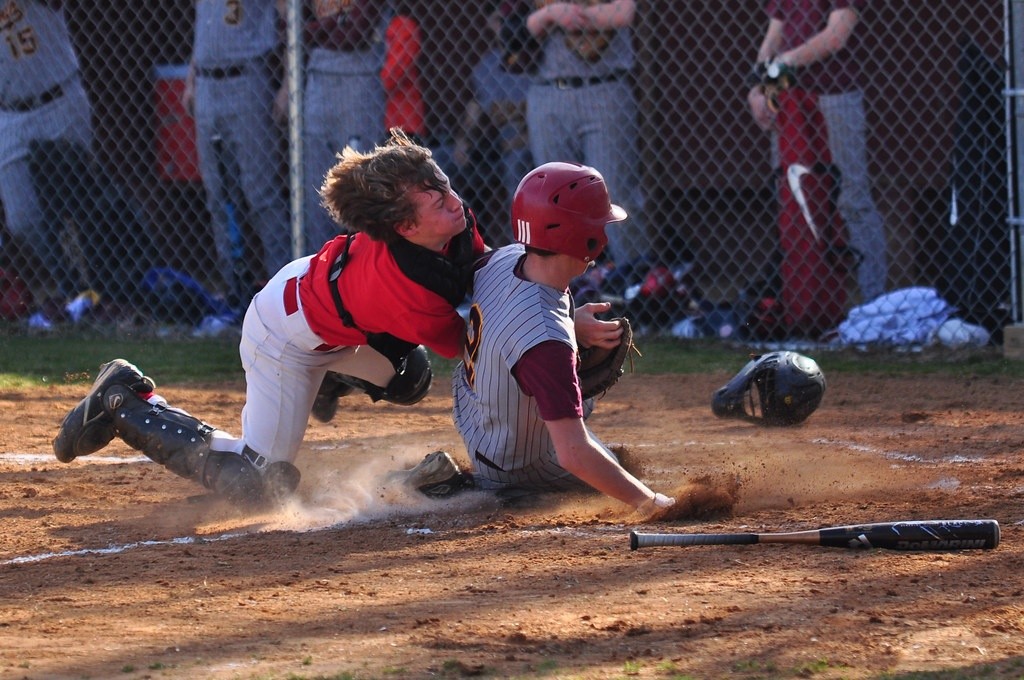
[627,518,1002,552]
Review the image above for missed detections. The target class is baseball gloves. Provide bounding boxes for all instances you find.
[577,316,643,402]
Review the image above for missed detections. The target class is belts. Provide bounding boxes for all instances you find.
[193,65,242,79]
[1,84,63,112]
[283,277,299,317]
[542,75,622,89]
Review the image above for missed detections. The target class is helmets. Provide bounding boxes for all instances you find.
[511,161,628,262]
[710,350,826,426]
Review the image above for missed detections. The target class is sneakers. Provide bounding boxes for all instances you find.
[311,370,339,424]
[52,359,156,464]
[382,451,473,501]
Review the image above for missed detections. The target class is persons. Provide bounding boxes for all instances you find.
[747,1,886,310]
[271,0,424,258]
[394,163,675,516]
[526,0,640,268]
[431,0,534,236]
[53,127,624,513]
[183,0,289,337]
[0,0,102,336]
